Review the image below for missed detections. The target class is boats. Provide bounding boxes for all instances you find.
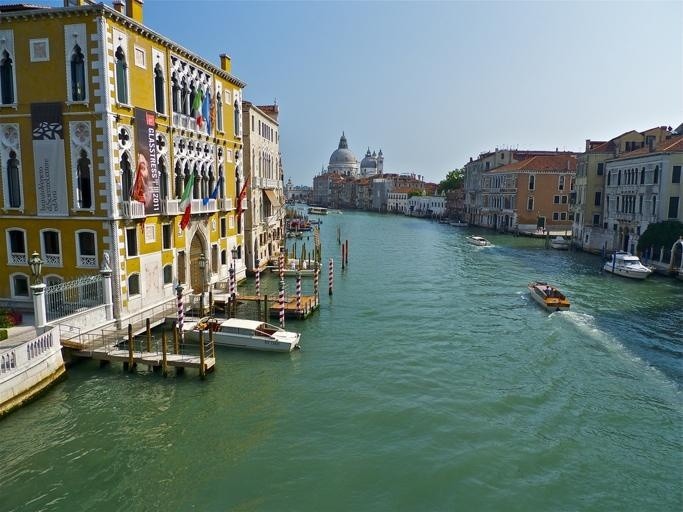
[440,218,470,227]
[270,260,322,277]
[176,317,301,352]
[526,279,571,313]
[465,234,491,247]
[550,236,569,251]
[285,199,328,240]
[604,249,652,281]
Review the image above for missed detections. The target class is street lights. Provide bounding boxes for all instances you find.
[198,252,209,296]
[28,249,43,284]
[231,244,237,283]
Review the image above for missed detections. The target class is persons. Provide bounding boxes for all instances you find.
[549,287,554,298]
[543,285,549,298]
[300,259,306,270]
[290,260,294,270]
[131,161,151,209]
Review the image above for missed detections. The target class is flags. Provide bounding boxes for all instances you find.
[192,85,202,129]
[236,177,248,221]
[201,87,211,135]
[177,170,193,230]
